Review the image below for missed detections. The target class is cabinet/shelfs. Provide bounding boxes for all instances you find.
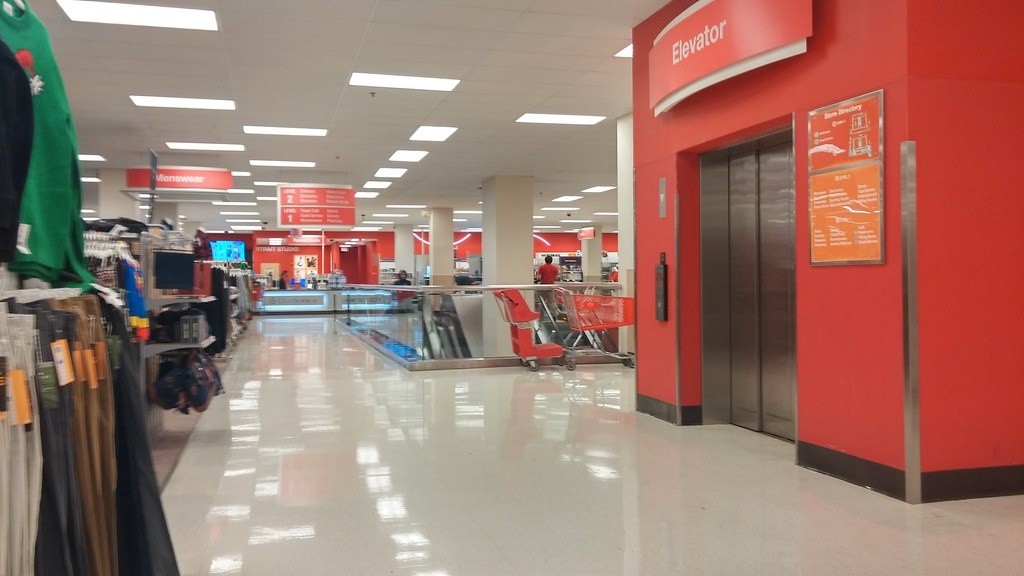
[120,230,219,448]
[379,268,413,286]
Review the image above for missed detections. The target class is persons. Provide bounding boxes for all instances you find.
[194,227,213,260]
[297,257,303,266]
[307,257,317,267]
[226,245,240,260]
[278,272,292,289]
[393,270,411,285]
[537,256,557,323]
[609,267,618,294]
[309,270,315,275]
[296,270,302,280]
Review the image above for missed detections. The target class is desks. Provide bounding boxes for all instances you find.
[258,286,393,318]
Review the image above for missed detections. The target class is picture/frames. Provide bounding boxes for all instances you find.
[259,262,280,281]
[293,254,318,284]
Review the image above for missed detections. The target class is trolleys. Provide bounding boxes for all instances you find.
[494,288,565,371]
[551,287,635,371]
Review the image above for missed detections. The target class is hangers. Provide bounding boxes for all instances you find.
[0,233,140,310]
[208,260,270,287]
